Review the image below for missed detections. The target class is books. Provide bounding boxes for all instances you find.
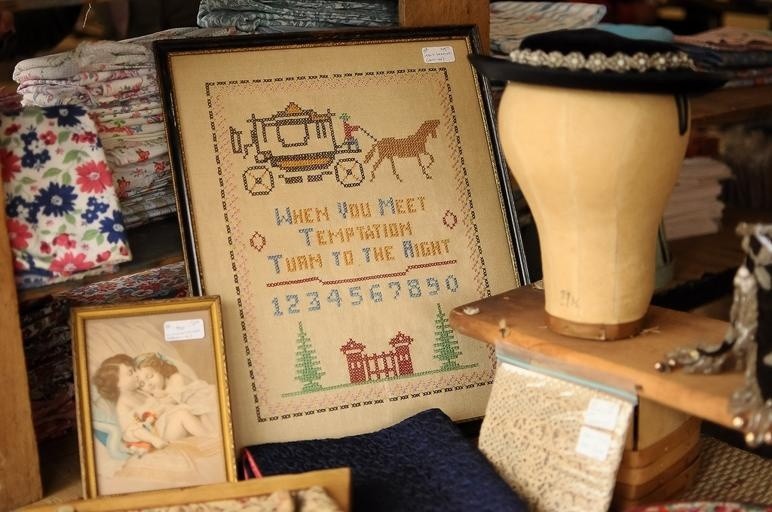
[661,155,734,241]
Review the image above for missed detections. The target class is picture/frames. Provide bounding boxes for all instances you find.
[149,17,540,448]
[65,293,241,505]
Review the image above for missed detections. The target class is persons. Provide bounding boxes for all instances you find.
[93,353,220,459]
